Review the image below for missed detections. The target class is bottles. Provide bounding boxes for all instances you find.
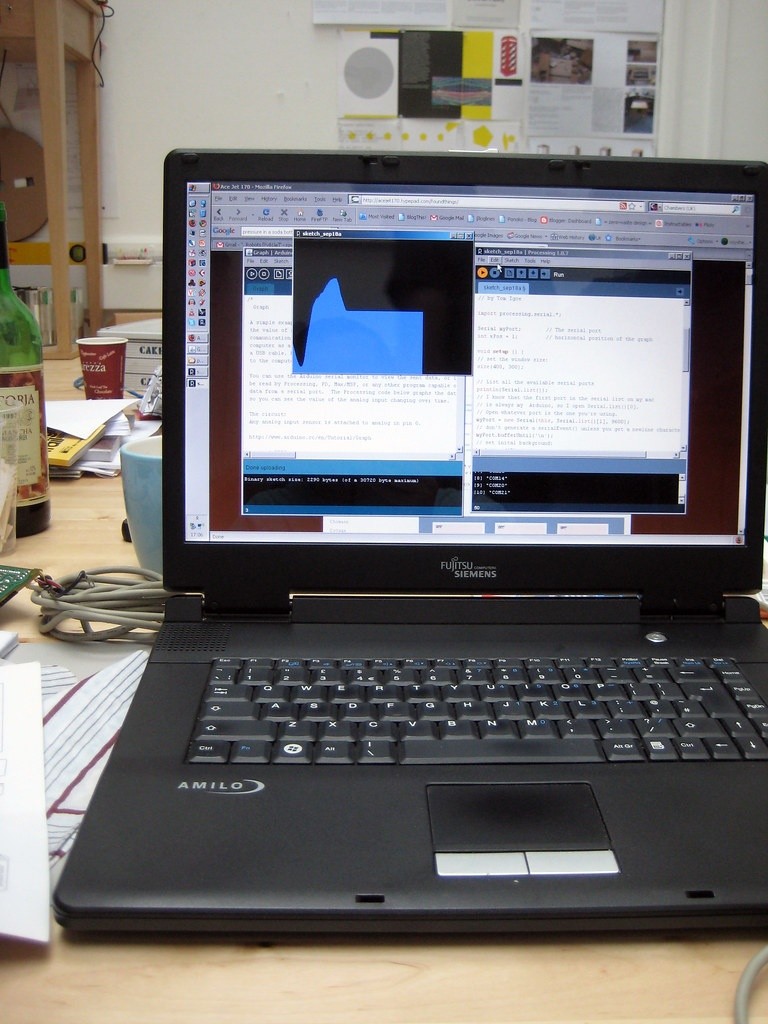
[0,203,50,538]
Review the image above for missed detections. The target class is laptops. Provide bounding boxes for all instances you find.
[51,150,768,944]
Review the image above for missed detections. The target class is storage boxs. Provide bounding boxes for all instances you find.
[96,318,164,393]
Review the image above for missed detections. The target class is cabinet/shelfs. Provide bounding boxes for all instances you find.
[0,1,106,364]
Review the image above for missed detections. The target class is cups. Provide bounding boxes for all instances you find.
[121,435,161,580]
[76,338,129,401]
[0,399,22,558]
